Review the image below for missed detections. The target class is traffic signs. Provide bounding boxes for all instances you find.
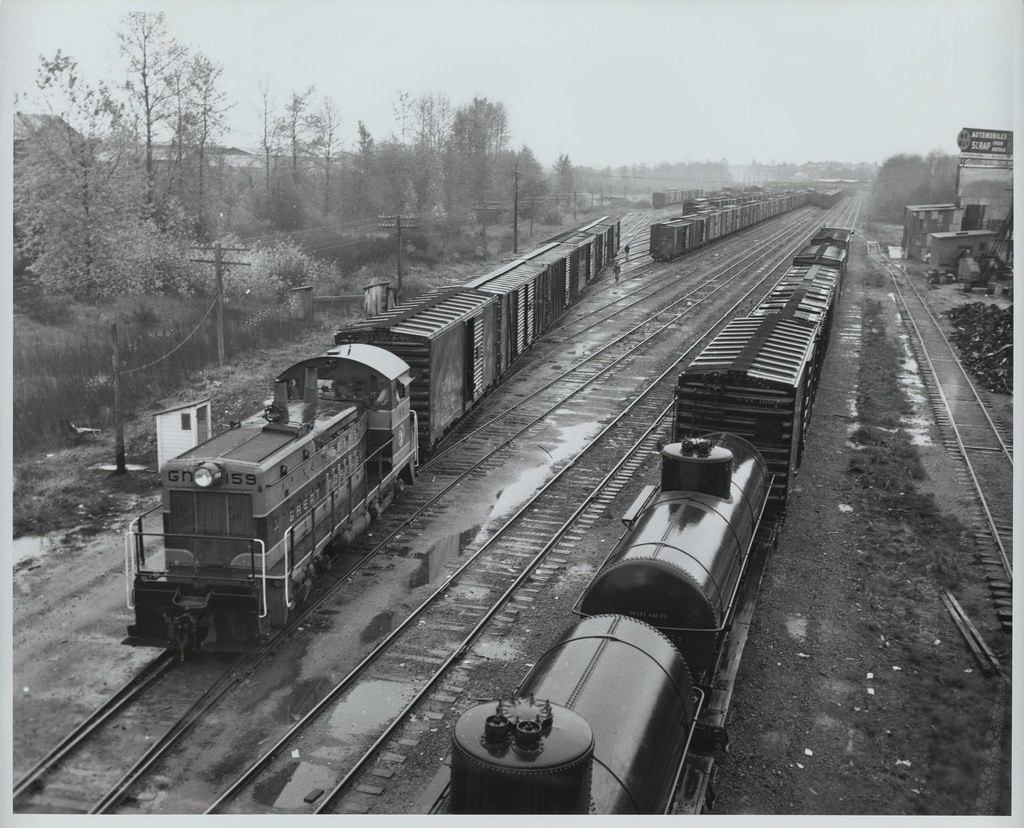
[957,127,1014,160]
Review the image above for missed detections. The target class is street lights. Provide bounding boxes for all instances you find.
[513,170,522,252]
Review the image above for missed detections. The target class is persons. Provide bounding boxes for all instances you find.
[624,244,630,261]
[612,262,621,283]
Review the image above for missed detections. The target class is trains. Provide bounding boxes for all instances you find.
[647,175,852,262]
[125,217,633,659]
[449,225,868,814]
